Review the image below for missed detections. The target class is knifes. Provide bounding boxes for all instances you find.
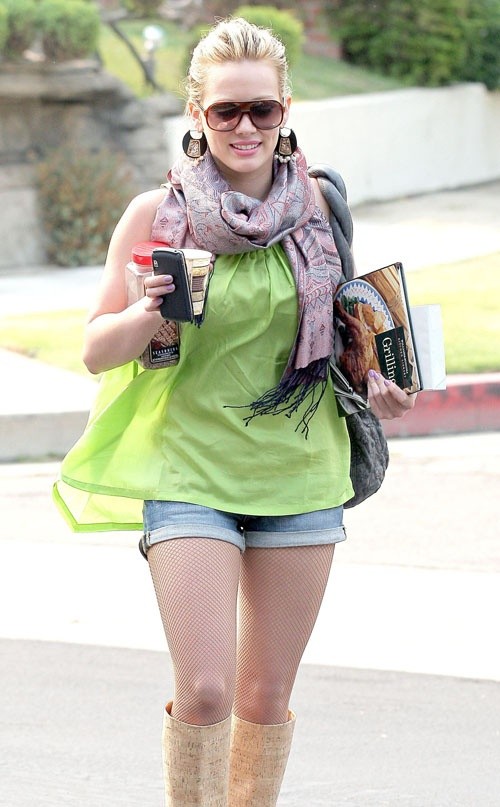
[329,361,366,410]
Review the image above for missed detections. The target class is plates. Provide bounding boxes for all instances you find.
[328,278,399,413]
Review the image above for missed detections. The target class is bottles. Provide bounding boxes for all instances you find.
[123,241,181,370]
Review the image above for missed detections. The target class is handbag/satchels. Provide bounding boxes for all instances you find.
[343,411,390,509]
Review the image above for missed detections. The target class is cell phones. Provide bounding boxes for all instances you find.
[151,247,195,322]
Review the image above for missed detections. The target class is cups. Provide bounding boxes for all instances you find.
[171,246,212,316]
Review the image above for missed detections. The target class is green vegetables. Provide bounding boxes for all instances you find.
[341,295,360,318]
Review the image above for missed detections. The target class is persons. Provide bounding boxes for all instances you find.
[81,16,422,807]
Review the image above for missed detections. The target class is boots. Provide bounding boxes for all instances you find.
[229,707,296,807]
[162,701,230,807]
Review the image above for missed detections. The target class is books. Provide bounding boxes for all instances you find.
[324,262,423,417]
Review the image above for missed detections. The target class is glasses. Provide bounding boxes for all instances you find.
[196,99,284,131]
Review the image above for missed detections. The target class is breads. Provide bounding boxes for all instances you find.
[354,302,387,333]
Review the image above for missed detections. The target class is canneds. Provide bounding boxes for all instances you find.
[124,241,181,370]
